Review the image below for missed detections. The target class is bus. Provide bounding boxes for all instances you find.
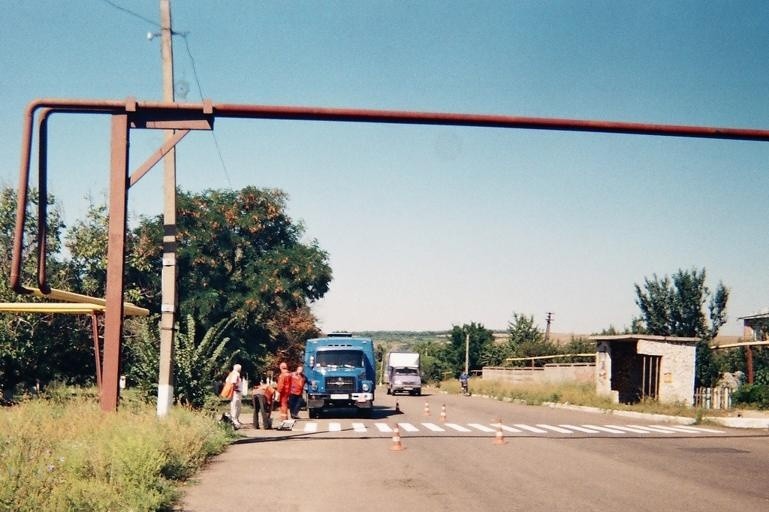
[472,369,482,379]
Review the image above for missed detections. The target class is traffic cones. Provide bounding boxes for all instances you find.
[493,414,509,447]
[423,400,432,417]
[439,403,448,422]
[388,423,406,452]
[395,400,401,413]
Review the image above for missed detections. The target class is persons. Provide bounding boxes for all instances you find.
[274,362,291,421]
[459,371,470,394]
[225,364,247,429]
[251,385,275,430]
[288,366,306,420]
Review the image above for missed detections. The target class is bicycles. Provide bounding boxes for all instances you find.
[459,379,469,395]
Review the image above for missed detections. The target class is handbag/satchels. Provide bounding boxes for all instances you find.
[221,384,233,400]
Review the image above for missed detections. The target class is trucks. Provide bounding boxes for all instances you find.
[382,352,423,396]
[305,332,377,419]
